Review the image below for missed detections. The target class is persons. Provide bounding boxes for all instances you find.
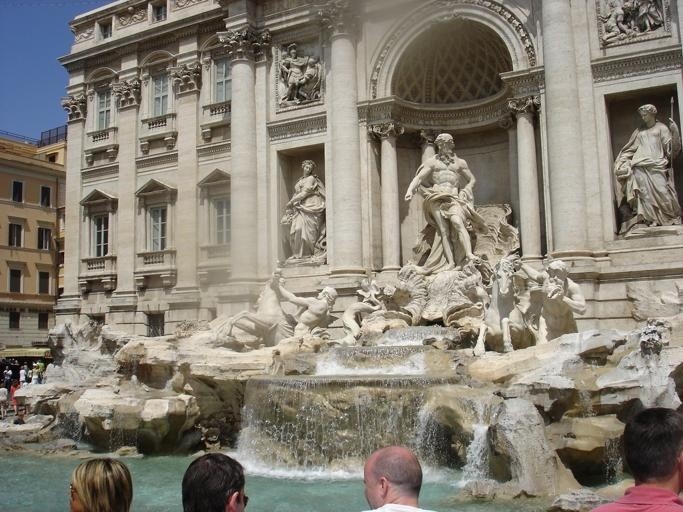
[514,259,586,344]
[280,49,305,102]
[285,160,326,260]
[181,453,248,512]
[613,103,683,234]
[296,56,322,104]
[361,446,438,512]
[405,133,482,270]
[633,0,663,33]
[69,458,133,512]
[0,358,46,419]
[278,278,338,340]
[602,2,634,41]
[590,407,683,512]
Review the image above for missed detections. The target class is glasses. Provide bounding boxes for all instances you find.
[243,494,249,508]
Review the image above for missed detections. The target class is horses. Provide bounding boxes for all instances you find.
[467,245,525,357]
[224,266,295,348]
